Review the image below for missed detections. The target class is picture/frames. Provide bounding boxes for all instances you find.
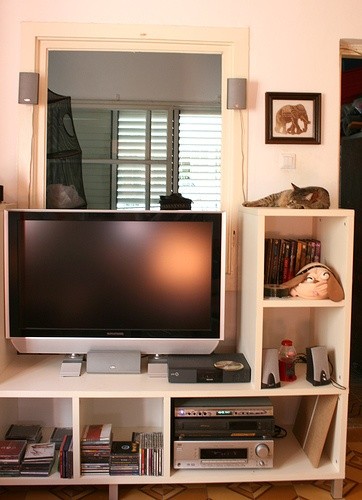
[263,92,322,145]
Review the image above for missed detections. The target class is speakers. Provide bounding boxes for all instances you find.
[261,346,282,388]
[226,76,247,112]
[305,344,333,386]
[17,70,41,105]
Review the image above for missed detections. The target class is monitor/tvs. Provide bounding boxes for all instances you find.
[2,207,227,377]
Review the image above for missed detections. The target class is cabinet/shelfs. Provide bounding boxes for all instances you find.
[0,206,356,499]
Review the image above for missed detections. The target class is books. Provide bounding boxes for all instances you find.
[0,423,73,479]
[80,423,162,476]
[264,238,321,297]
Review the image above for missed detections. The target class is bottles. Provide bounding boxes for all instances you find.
[278,339,296,382]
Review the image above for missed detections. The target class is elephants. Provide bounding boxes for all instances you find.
[274,104,311,135]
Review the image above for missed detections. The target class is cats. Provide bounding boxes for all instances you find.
[242,182,330,209]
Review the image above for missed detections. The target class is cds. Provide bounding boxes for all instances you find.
[264,283,291,297]
[214,359,245,371]
